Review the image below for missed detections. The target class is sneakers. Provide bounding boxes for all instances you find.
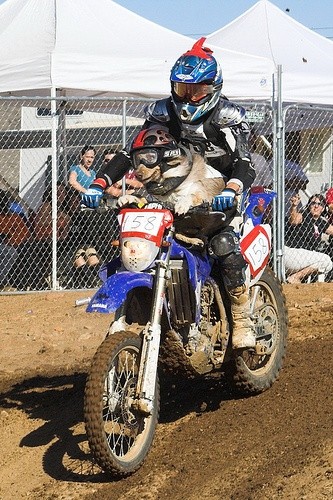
[231,304,258,349]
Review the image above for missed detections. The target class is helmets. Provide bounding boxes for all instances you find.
[130,128,178,154]
[169,36,223,126]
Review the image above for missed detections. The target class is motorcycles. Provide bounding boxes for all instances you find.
[81,189,289,477]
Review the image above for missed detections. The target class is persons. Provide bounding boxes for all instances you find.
[69,146,96,198]
[287,193,332,283]
[0,182,99,287]
[99,148,143,198]
[79,50,255,349]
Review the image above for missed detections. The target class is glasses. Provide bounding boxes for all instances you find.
[131,147,181,170]
[309,202,324,207]
[171,79,223,106]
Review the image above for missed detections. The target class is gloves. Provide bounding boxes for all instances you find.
[81,187,104,209]
[212,188,236,212]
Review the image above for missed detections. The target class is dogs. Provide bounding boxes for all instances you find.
[115,144,237,248]
